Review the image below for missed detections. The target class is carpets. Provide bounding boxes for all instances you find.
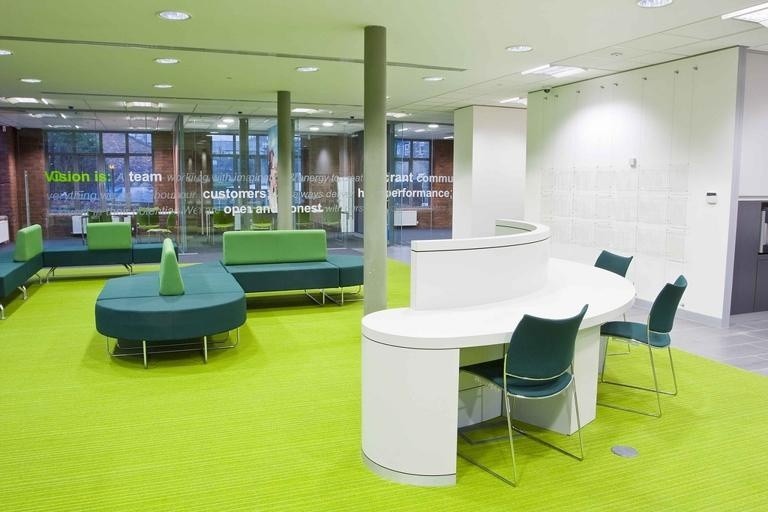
[0,248,768,511]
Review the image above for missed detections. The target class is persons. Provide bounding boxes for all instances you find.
[267,148,278,212]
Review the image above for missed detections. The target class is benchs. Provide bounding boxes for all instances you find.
[39,220,179,281]
[97,227,248,370]
[217,227,368,309]
[1,224,44,317]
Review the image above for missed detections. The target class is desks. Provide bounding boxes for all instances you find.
[356,257,640,491]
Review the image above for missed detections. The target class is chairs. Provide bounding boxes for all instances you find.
[71,204,346,246]
[459,301,593,488]
[596,271,690,421]
[590,249,637,284]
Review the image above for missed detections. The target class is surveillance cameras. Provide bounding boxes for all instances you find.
[543,87,551,94]
